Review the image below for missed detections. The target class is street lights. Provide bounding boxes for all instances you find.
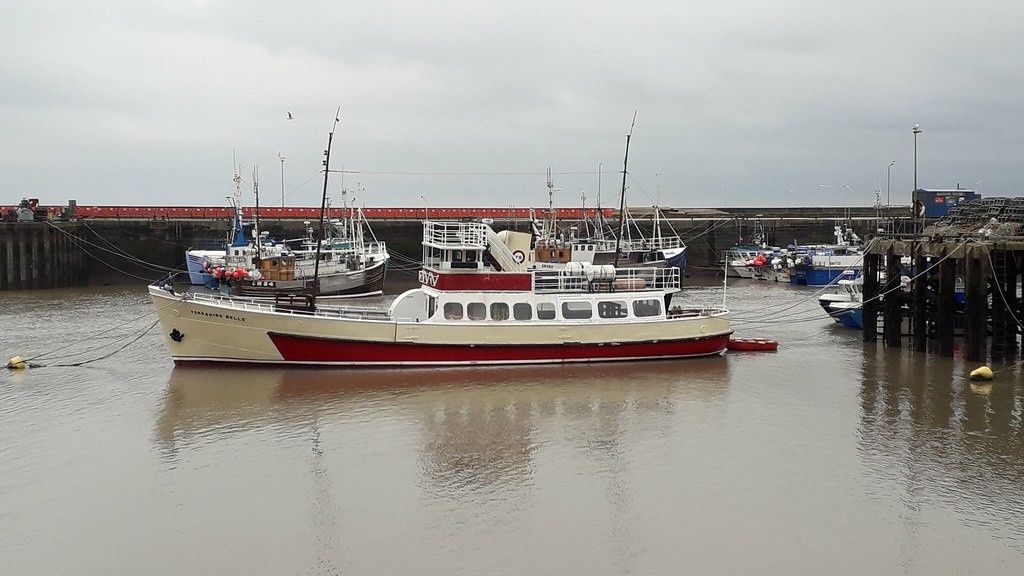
[888,163,894,210]
[912,123,923,217]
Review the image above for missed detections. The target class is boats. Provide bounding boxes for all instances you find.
[720,206,931,329]
[182,106,391,297]
[145,108,736,368]
